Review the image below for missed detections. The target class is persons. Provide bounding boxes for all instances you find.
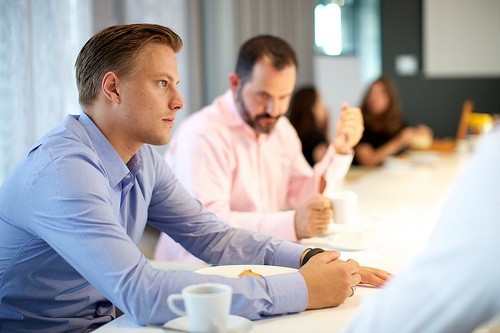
[292,84,327,167]
[154,34,364,263]
[0,24,391,333]
[352,77,431,166]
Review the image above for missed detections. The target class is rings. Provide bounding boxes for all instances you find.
[350,286,356,297]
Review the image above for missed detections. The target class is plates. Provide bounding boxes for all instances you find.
[163,315,253,333]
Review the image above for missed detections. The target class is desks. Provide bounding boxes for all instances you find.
[89,123,500,333]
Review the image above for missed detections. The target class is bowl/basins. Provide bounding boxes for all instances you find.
[195,264,298,279]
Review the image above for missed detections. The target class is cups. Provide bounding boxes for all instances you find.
[329,190,358,224]
[167,284,232,332]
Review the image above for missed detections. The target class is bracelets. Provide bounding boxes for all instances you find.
[302,248,325,265]
